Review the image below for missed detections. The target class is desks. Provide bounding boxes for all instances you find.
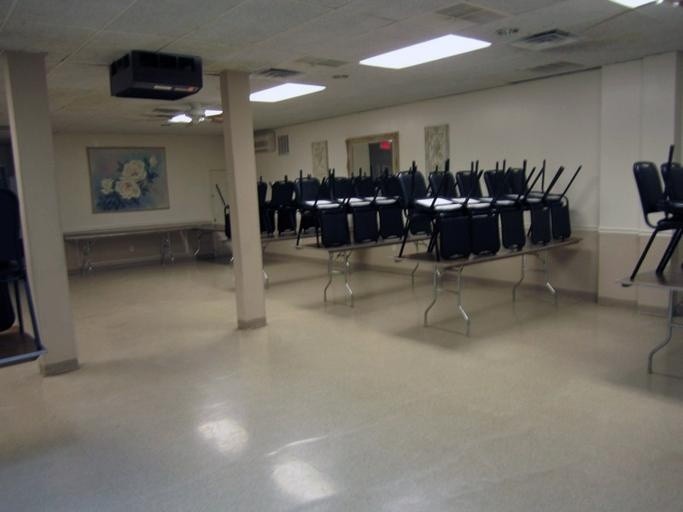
[618,267,682,375]
[62,221,223,279]
[220,234,583,337]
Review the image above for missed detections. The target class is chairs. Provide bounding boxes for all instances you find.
[210,156,584,259]
[627,159,681,283]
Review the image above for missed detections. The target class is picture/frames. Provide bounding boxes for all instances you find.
[85,144,170,214]
